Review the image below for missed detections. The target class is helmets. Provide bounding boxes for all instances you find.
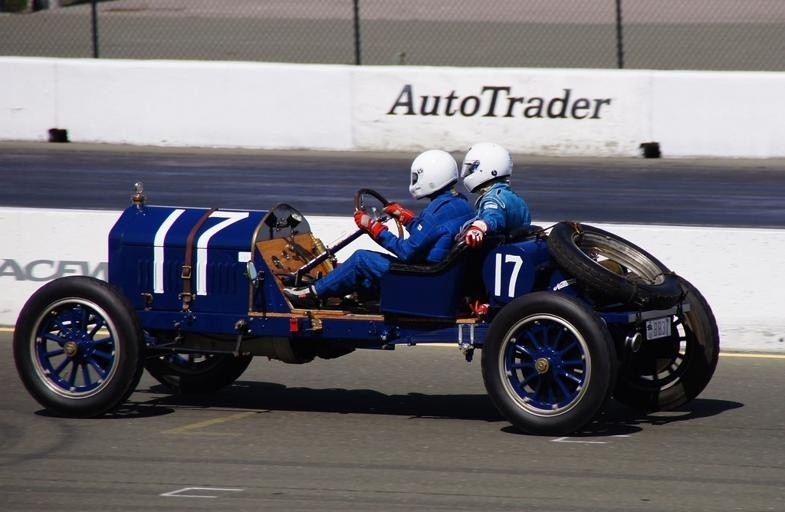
[409,150,458,199]
[461,144,513,192]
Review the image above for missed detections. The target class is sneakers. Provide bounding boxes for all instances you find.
[284,287,316,307]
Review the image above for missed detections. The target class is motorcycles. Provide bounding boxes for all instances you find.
[12,181,720,437]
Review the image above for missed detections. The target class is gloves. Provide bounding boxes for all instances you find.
[354,210,388,238]
[384,203,415,223]
[465,220,487,247]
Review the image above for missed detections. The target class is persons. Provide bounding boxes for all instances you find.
[282,149,476,309]
[460,142,532,248]
